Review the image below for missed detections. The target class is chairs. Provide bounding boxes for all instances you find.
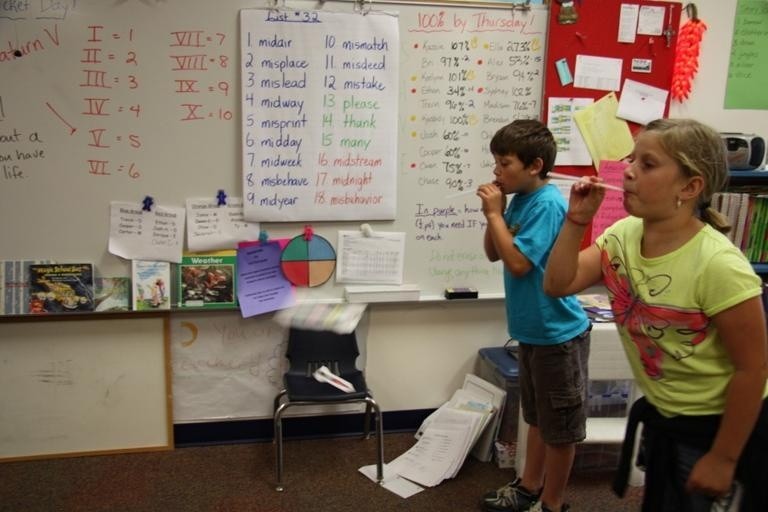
[271,309,385,491]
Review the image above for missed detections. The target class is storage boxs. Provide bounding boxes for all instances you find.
[477,346,519,429]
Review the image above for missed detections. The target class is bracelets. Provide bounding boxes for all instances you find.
[707,447,740,465]
[563,213,591,227]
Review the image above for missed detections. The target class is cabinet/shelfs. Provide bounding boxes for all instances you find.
[694,170,768,274]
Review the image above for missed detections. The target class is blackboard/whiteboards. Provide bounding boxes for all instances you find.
[0,1,550,323]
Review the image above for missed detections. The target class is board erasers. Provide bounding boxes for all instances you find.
[445,288,479,299]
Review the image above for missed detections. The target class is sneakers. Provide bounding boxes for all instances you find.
[483,477,569,512]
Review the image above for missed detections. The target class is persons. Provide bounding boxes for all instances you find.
[542,117,768,511]
[474,117,593,512]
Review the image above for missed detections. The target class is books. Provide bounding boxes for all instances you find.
[95,274,129,312]
[453,369,507,466]
[706,190,768,265]
[176,247,240,309]
[131,258,171,312]
[577,292,616,324]
[3,257,57,315]
[26,262,97,313]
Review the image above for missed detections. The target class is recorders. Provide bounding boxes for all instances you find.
[718,132,765,171]
